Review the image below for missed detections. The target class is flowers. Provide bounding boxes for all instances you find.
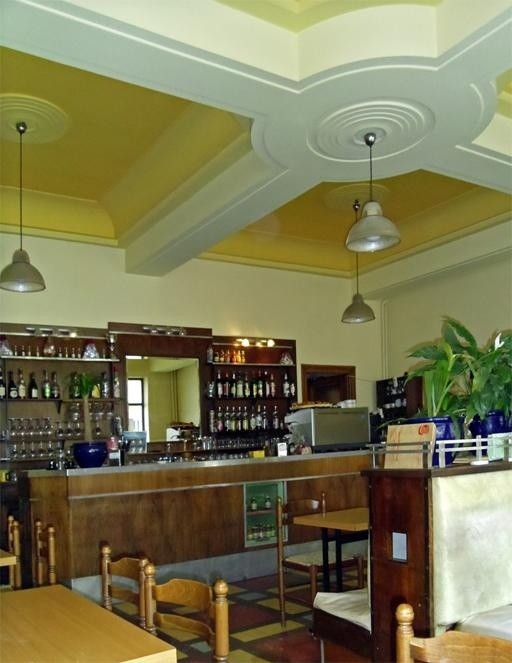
[375,334,505,456]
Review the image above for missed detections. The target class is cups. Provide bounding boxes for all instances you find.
[5,470,17,481]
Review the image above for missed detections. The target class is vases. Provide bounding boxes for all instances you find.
[400,416,464,466]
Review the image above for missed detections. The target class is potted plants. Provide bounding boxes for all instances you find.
[404,315,512,456]
[65,372,109,469]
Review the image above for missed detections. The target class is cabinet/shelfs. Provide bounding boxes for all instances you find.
[245,483,277,542]
[212,342,296,452]
[376,376,422,422]
[0,355,123,460]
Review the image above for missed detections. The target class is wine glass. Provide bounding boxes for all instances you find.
[8,410,104,459]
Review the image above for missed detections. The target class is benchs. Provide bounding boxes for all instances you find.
[455,604,511,639]
[313,588,373,662]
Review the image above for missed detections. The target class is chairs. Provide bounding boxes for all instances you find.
[102,546,148,629]
[395,604,512,663]
[0,516,22,591]
[35,522,56,586]
[276,492,364,640]
[144,563,229,663]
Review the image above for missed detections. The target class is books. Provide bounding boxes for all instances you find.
[384,422,436,469]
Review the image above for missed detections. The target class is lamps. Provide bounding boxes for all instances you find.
[345,133,401,254]
[1,123,46,292]
[341,200,374,324]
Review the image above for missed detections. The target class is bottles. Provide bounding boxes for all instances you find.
[102,347,106,358]
[156,451,249,463]
[48,458,74,470]
[120,437,144,454]
[0,366,109,400]
[382,387,406,408]
[214,403,280,431]
[205,343,248,363]
[190,434,270,449]
[213,369,291,400]
[14,344,82,358]
[246,494,278,541]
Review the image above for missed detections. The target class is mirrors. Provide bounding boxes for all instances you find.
[126,355,200,442]
